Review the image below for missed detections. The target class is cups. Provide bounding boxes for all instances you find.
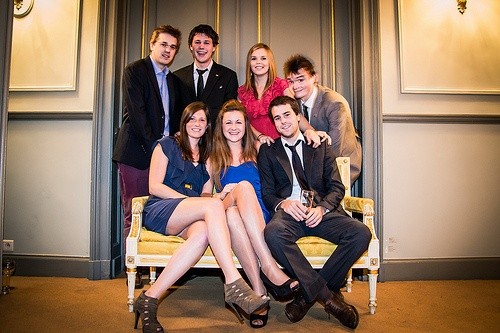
[2,264,10,294]
[301,189,315,215]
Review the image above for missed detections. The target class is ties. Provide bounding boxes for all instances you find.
[302,103,309,123]
[196,68,208,101]
[285,139,310,191]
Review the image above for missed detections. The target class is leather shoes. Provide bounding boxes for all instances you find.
[325,290,359,329]
[284,296,318,323]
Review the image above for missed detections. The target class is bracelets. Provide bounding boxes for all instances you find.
[257,134,265,140]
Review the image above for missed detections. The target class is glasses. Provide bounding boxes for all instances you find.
[156,41,179,51]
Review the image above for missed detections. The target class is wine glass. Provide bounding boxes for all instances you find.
[2,259,16,290]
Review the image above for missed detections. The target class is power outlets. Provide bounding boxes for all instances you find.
[386,235,397,254]
[2,240,14,252]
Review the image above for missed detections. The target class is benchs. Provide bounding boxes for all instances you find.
[125,157,381,316]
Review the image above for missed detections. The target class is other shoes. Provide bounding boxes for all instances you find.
[126,272,143,288]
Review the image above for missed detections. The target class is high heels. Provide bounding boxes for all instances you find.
[224,277,271,321]
[260,267,301,302]
[132,290,164,333]
[250,302,269,327]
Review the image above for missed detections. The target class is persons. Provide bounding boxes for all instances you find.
[283,55,363,187]
[203,101,301,330]
[258,97,375,328]
[113,24,186,288]
[172,25,238,135]
[131,102,272,333]
[236,43,333,149]
